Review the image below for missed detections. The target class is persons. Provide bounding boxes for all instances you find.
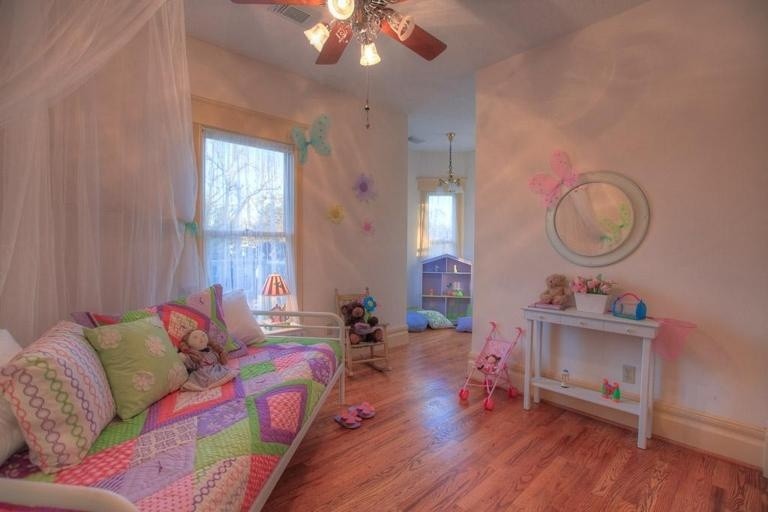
[175,327,239,394]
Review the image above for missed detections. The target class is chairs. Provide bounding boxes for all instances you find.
[334,286,394,376]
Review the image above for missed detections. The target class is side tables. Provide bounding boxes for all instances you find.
[258,320,305,337]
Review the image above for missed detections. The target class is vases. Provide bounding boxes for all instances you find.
[575,291,612,313]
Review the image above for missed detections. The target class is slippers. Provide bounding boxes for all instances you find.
[335,412,360,430]
[349,402,375,419]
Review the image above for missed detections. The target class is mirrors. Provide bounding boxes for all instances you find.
[546,169,650,268]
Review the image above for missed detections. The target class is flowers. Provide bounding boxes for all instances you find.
[570,274,621,296]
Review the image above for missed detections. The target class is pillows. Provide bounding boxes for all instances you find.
[407,310,472,333]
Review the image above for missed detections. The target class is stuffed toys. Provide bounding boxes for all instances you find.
[339,300,384,344]
[540,272,573,305]
[476,353,499,371]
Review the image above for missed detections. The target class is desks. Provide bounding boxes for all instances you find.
[522,305,661,450]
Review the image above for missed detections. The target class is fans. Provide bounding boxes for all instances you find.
[231,0,447,66]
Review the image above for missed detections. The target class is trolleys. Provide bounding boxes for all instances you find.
[456,320,523,412]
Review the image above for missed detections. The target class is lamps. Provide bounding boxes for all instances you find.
[438,131,461,193]
[262,274,290,321]
[303,0,416,65]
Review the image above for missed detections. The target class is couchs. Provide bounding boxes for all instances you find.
[0,311,346,512]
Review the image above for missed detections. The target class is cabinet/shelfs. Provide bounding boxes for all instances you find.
[420,254,471,322]
